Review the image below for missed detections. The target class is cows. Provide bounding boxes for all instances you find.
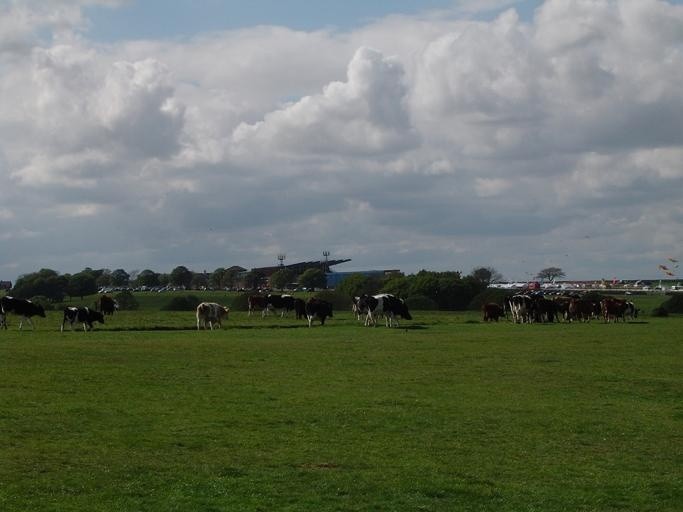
[0,294,48,330]
[351,292,413,329]
[98,294,118,316]
[504,292,640,324]
[247,293,334,328]
[196,303,230,331]
[60,306,105,333]
[480,302,506,323]
